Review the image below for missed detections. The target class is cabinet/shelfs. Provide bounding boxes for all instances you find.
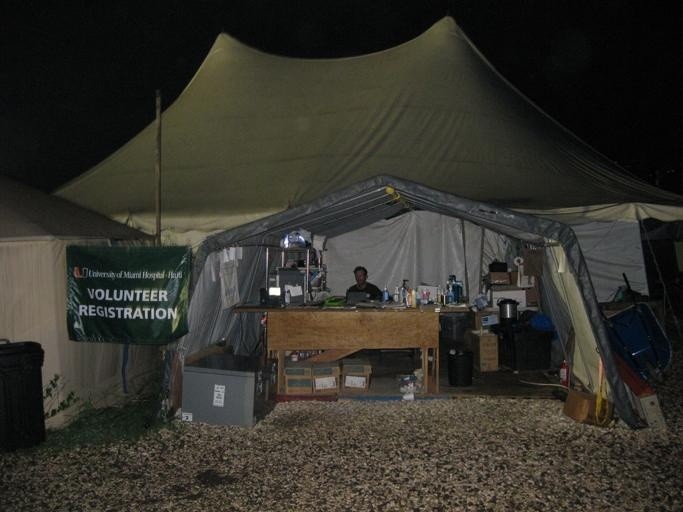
[265,248,324,307]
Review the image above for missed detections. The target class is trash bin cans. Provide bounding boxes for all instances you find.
[0,338,46,453]
[447,351,473,386]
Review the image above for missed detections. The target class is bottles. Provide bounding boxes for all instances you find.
[383,275,464,308]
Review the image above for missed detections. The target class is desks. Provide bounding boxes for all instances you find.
[233,298,473,397]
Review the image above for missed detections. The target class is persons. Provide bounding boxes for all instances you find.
[345,266,392,302]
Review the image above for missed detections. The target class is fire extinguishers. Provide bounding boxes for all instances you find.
[559,360,569,390]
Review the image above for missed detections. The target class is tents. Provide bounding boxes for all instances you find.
[160,174,647,435]
[0,175,153,431]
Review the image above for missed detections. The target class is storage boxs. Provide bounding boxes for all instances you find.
[463,247,544,372]
[181,355,279,431]
[283,358,373,395]
